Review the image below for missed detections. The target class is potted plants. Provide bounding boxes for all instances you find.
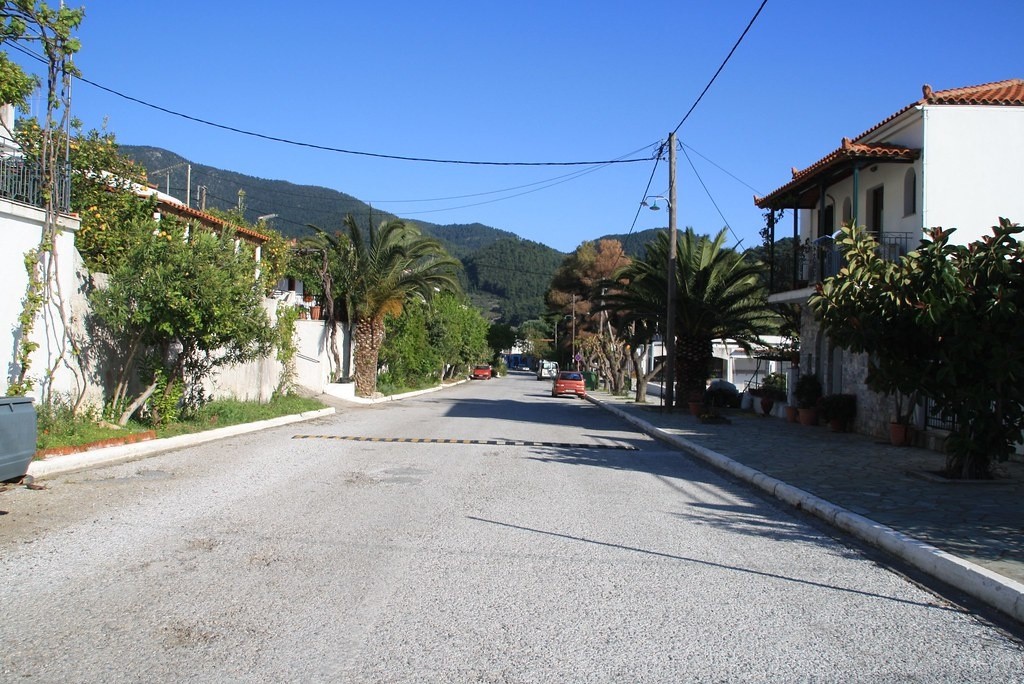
[822,392,850,433]
[804,217,970,449]
[792,373,824,427]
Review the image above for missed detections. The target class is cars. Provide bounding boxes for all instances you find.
[473,364,491,379]
[551,370,586,400]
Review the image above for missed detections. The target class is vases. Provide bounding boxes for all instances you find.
[784,406,795,422]
[687,401,703,416]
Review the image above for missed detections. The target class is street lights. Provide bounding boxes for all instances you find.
[564,315,574,371]
[640,195,677,411]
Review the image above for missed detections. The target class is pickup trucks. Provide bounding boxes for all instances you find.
[516,363,530,371]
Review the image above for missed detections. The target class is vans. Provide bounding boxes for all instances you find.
[536,361,559,381]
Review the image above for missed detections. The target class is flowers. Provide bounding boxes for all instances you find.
[687,391,703,402]
